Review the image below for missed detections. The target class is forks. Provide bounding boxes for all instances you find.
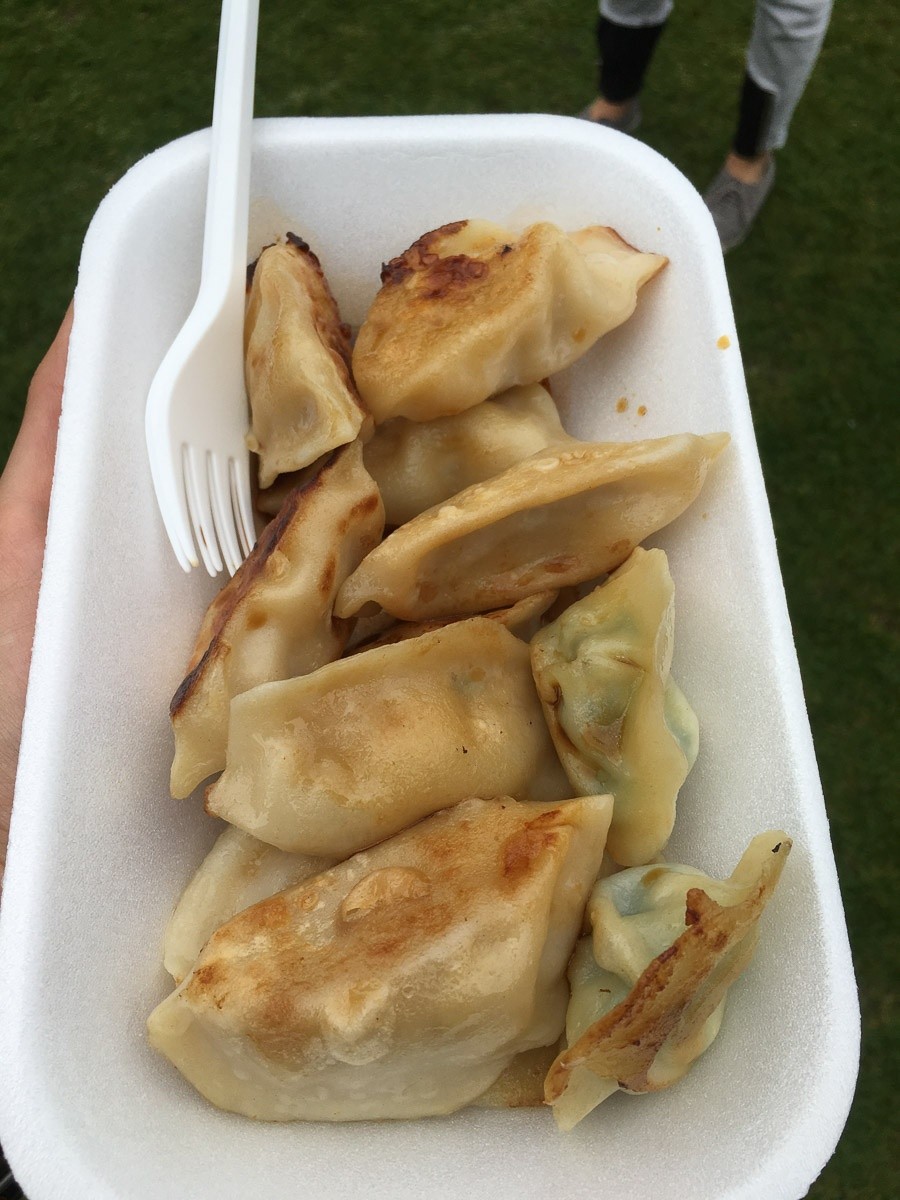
[146,0,260,577]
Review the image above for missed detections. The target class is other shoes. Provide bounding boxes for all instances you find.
[699,155,779,252]
[572,100,635,132]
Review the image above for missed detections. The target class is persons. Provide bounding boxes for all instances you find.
[567,0,835,256]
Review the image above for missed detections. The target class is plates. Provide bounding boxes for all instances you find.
[2,113,863,1194]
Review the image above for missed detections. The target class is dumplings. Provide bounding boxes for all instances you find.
[137,223,794,1137]
